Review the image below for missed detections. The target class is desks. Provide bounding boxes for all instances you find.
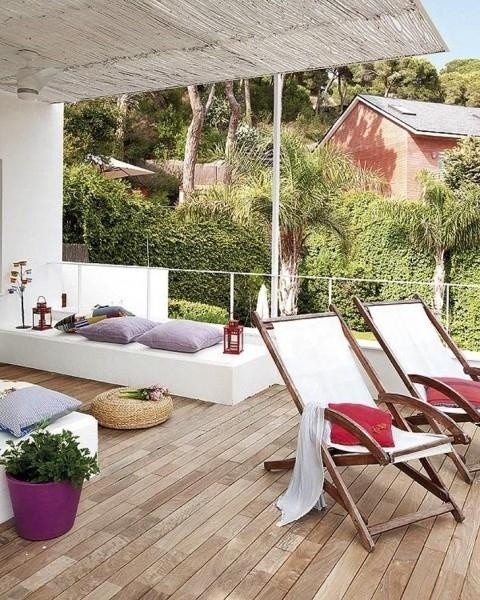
[0,313,276,407]
[0,378,99,526]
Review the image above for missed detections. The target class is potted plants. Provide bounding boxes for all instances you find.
[0,418,102,541]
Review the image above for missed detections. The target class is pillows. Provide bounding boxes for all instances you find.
[0,385,82,434]
[54,307,225,354]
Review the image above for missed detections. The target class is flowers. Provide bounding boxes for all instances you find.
[119,383,171,402]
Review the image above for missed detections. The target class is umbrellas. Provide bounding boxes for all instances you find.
[87,151,155,185]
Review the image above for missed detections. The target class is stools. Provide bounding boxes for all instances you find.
[89,386,173,430]
